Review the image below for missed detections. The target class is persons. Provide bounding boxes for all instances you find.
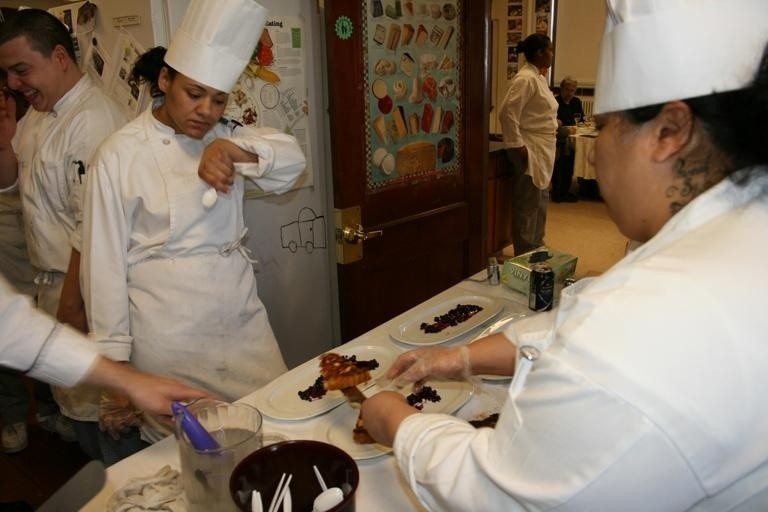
[499,32,560,260]
[1,9,210,462]
[363,1,768,512]
[549,77,586,203]
[78,0,305,449]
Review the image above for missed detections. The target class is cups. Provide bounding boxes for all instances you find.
[174,402,262,511]
[231,440,359,511]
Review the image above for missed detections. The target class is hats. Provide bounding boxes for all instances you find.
[163,0,269,94]
[590,0,768,118]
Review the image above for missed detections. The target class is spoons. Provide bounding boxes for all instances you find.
[201,187,219,208]
[309,484,344,511]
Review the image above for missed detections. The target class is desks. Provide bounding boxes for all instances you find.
[490,141,512,253]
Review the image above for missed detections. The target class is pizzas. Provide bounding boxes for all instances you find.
[319,354,371,389]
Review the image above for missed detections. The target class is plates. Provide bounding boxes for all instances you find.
[324,376,475,463]
[260,342,401,422]
[389,292,507,348]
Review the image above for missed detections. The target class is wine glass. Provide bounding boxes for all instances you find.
[573,113,592,129]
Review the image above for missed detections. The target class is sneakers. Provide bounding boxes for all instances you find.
[0,412,48,454]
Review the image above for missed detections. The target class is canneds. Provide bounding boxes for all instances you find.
[529,266,554,312]
